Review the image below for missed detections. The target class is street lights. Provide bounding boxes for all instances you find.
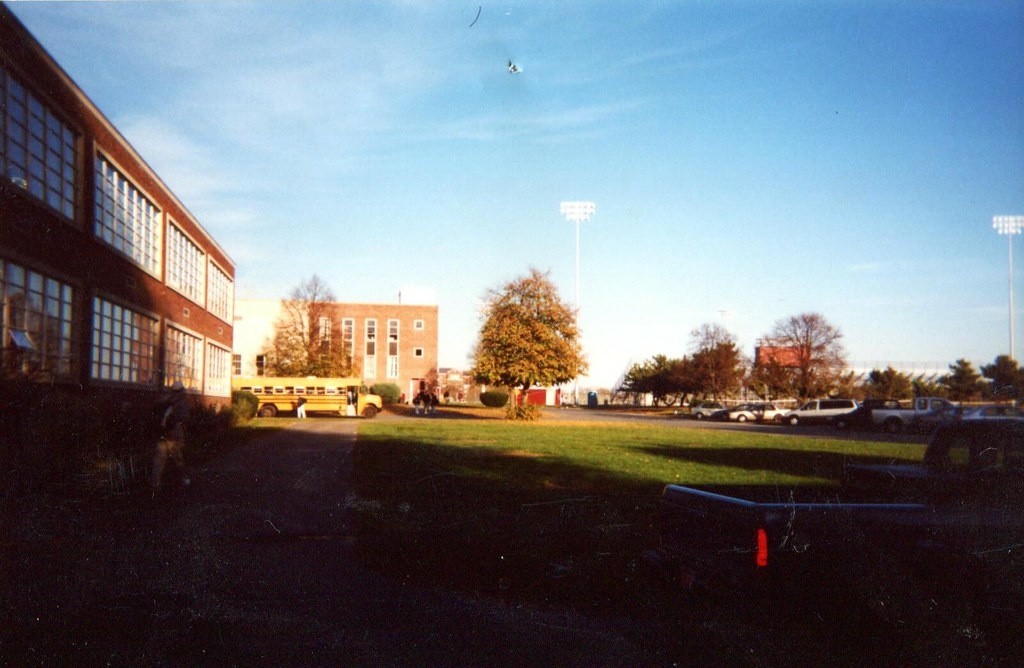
[994,214,1024,360]
[561,201,597,405]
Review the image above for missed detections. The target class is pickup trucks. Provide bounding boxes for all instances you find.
[837,396,956,433]
[658,416,1024,614]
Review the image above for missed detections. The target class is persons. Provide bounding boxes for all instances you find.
[423,392,430,415]
[430,393,436,414]
[296,398,306,418]
[414,393,420,415]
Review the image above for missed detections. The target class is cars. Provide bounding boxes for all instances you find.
[721,402,791,422]
[957,404,1024,423]
[691,403,726,420]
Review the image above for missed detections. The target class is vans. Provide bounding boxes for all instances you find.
[783,399,862,429]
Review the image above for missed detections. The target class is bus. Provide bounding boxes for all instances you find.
[232,376,383,418]
